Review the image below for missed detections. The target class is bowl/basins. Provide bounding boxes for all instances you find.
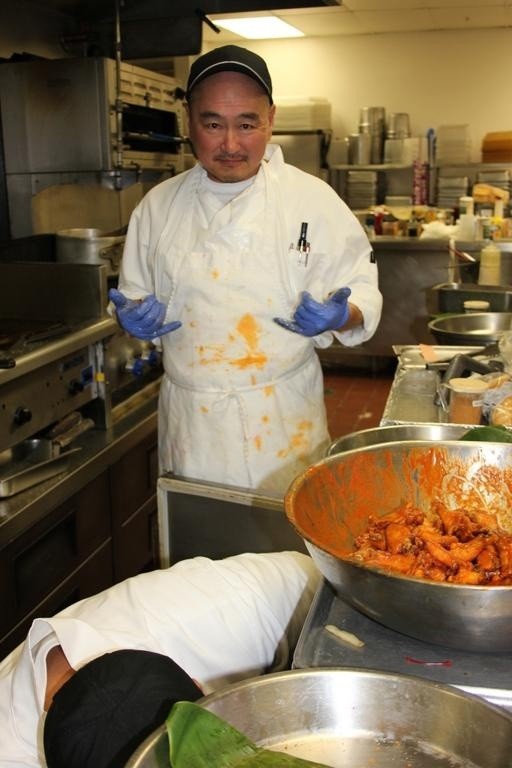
[427,312,512,346]
[359,106,410,166]
[326,421,486,456]
[282,440,511,659]
[123,669,509,767]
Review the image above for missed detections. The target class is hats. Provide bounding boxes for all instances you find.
[186,44,272,103]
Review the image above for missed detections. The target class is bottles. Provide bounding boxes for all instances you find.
[373,208,384,235]
[447,378,487,423]
[477,239,502,288]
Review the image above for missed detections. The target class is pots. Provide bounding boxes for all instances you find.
[54,225,127,280]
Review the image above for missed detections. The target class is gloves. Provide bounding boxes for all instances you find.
[109,285,182,342]
[273,286,351,337]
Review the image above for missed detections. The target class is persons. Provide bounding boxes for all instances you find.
[0,547,327,766]
[106,44,383,502]
